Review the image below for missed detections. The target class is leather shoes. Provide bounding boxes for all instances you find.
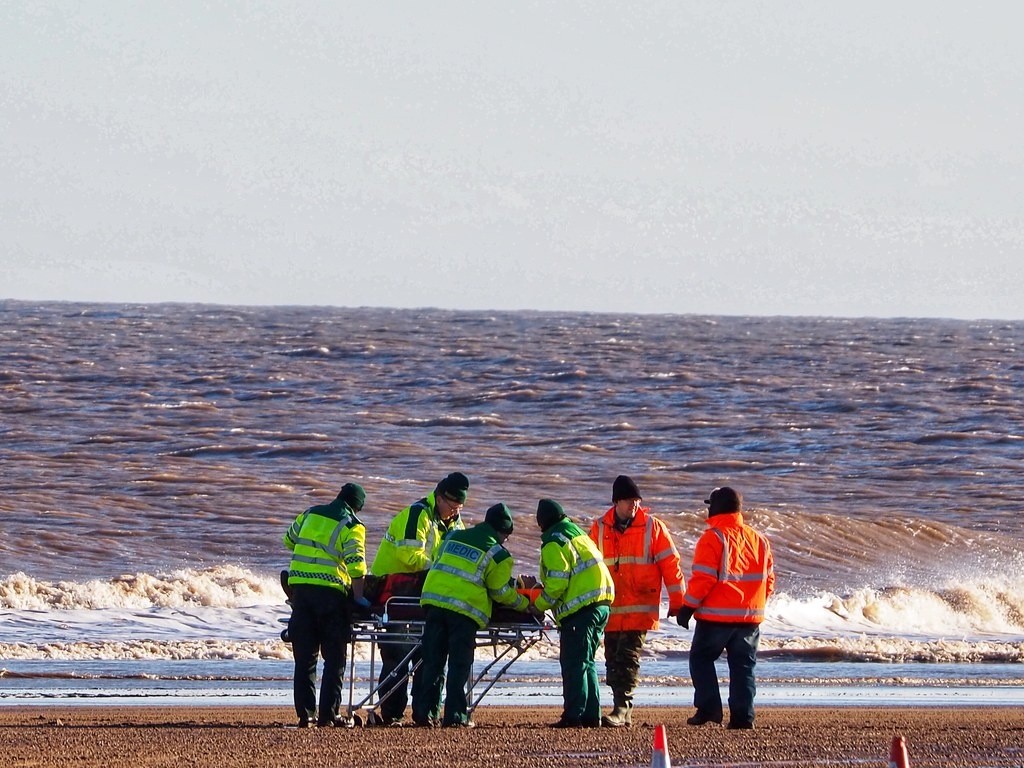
[299,717,318,728]
[318,715,355,728]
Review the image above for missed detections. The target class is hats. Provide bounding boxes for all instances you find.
[612,475,642,502]
[339,483,366,511]
[485,503,514,534]
[537,499,566,529]
[704,487,743,517]
[436,472,469,504]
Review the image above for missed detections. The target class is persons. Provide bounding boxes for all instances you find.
[529,499,615,729]
[280,570,544,606]
[283,482,367,727]
[410,502,531,728]
[677,486,775,729]
[588,475,686,726]
[371,471,469,727]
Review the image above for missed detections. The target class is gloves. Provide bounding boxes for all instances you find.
[667,611,675,618]
[677,606,696,630]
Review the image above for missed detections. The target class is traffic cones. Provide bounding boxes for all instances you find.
[887,735,908,768]
[650,724,672,768]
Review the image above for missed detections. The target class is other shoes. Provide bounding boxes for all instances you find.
[626,708,633,725]
[601,706,627,726]
[727,721,754,729]
[384,717,404,727]
[441,718,476,729]
[434,718,441,728]
[549,718,582,729]
[416,717,434,727]
[687,710,723,725]
[281,571,292,600]
[583,718,602,728]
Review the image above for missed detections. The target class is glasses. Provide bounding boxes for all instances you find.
[504,536,509,542]
[443,498,463,512]
